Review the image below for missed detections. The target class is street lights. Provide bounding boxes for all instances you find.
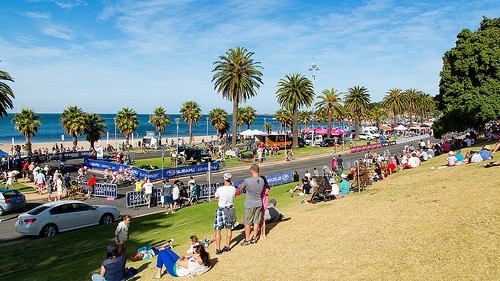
[114,116,117,150]
[308,57,320,100]
[175,118,180,168]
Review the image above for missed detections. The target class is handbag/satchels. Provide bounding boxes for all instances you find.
[224,208,237,223]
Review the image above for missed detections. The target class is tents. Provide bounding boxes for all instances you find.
[238,122,430,140]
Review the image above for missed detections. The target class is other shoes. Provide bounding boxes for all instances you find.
[152,274,161,279]
[172,211,176,214]
[250,235,258,244]
[169,238,174,246]
[216,249,221,255]
[166,213,168,214]
[169,246,174,249]
[240,239,250,246]
[221,246,231,252]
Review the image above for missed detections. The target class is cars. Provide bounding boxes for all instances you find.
[15,200,122,238]
[0,186,27,215]
[359,129,380,140]
[290,133,353,146]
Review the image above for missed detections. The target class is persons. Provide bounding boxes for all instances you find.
[130,238,173,262]
[257,176,271,239]
[239,164,264,246]
[285,121,500,204]
[91,244,126,281]
[151,244,211,279]
[115,214,131,258]
[214,172,236,254]
[0,138,279,215]
[231,199,282,231]
[186,236,215,253]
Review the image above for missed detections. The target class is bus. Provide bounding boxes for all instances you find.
[253,134,291,149]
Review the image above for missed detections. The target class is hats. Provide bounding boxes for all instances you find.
[449,151,454,155]
[268,199,277,205]
[340,174,347,178]
[223,173,232,180]
[250,164,259,170]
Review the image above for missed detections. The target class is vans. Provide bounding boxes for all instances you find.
[177,147,211,163]
